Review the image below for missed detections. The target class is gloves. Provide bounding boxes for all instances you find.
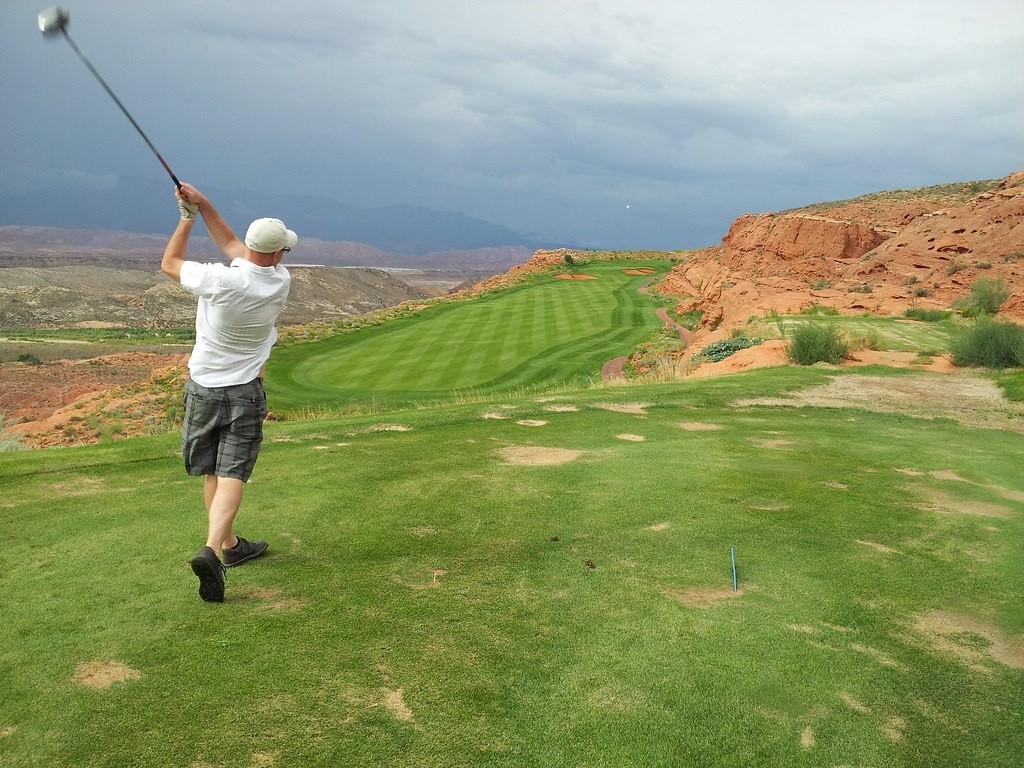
[177,199,199,223]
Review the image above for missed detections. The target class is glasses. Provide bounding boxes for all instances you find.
[273,246,290,254]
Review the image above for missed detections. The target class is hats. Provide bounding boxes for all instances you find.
[245,217,298,253]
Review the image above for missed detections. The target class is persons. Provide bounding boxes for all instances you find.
[161,182,298,604]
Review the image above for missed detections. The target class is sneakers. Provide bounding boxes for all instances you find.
[191,546,228,603]
[222,536,268,566]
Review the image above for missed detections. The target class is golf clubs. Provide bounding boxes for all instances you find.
[38,3,187,200]
